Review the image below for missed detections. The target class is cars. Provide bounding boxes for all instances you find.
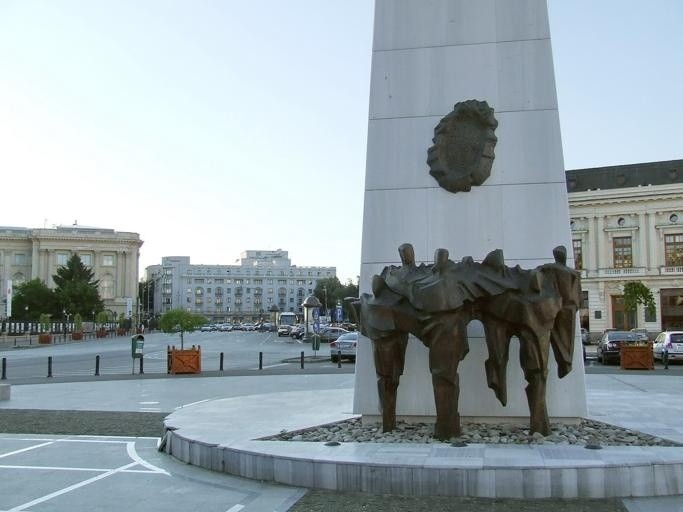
[581,325,682,368]
[189,308,358,365]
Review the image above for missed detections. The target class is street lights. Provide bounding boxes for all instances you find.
[22,304,119,334]
